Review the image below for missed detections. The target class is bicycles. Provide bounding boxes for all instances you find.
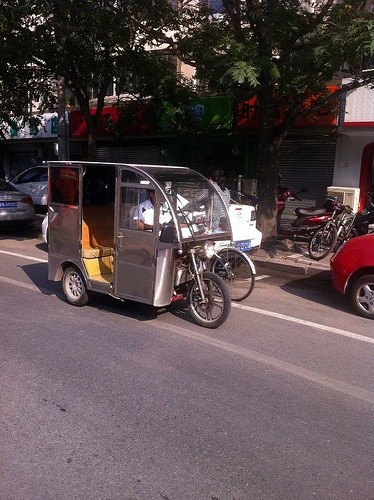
[203,240,257,301]
[308,196,360,260]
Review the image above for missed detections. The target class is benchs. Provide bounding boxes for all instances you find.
[82,206,114,258]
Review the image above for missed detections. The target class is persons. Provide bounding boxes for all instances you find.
[133,179,194,230]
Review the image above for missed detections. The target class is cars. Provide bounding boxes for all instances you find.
[42,181,262,271]
[329,233,374,320]
[0,178,35,226]
[10,166,48,214]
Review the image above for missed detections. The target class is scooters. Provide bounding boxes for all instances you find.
[277,172,352,246]
[352,192,374,236]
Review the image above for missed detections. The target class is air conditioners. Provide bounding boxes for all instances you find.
[232,178,257,196]
[326,186,360,214]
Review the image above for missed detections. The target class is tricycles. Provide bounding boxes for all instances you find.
[42,159,233,329]
